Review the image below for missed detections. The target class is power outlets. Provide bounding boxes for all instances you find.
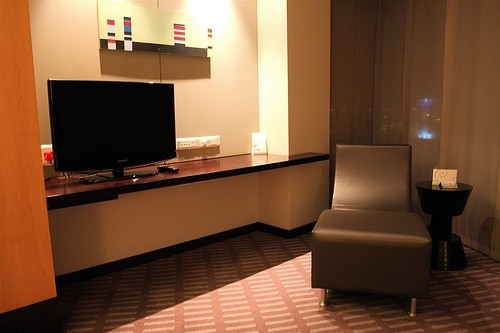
[196,136,220,148]
[41,144,54,167]
[175,138,196,151]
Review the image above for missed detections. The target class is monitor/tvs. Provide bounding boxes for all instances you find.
[46,77,176,185]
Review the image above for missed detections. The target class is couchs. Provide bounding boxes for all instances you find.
[310,145,433,318]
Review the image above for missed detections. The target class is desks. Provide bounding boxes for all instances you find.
[415,181,473,271]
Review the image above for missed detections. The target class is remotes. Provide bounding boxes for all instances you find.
[157,165,180,173]
